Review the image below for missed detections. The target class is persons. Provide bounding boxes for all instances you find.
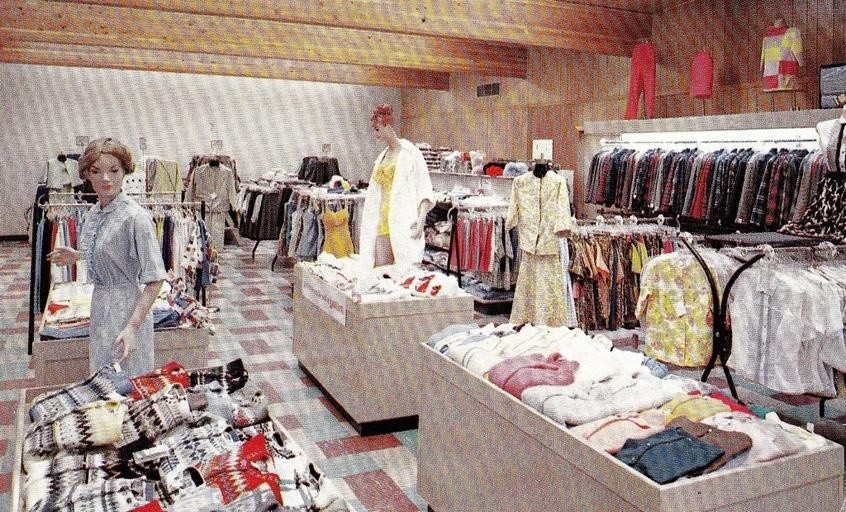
[759,18,808,91]
[802,106,846,242]
[46,137,167,378]
[690,54,714,100]
[361,102,436,268]
[625,38,655,118]
